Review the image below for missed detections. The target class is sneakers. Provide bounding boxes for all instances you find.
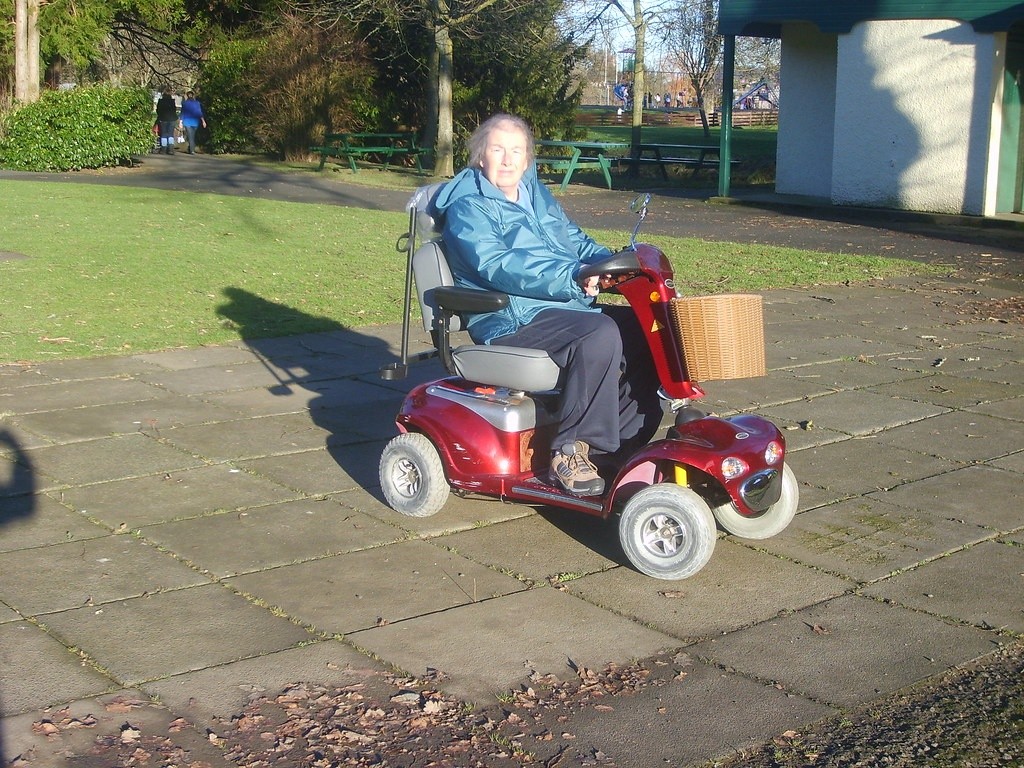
[548,441,605,497]
[606,443,644,465]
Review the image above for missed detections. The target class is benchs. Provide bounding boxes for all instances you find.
[306,146,433,157]
[535,155,743,169]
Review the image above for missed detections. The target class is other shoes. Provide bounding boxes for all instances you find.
[190,151,195,154]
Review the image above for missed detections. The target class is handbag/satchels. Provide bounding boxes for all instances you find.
[176,129,185,144]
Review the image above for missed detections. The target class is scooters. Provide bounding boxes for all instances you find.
[378,178,799,580]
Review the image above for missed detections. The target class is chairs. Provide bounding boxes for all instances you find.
[412,181,571,392]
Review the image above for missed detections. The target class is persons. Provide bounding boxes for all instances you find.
[688,95,693,106]
[156,89,177,155]
[643,92,671,109]
[179,91,207,155]
[621,84,633,110]
[436,114,664,498]
[677,97,681,107]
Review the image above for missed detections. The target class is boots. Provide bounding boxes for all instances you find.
[158,146,167,155]
[167,144,174,155]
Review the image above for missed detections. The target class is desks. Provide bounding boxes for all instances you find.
[540,141,629,193]
[625,144,720,183]
[315,132,424,176]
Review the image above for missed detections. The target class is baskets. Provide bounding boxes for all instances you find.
[668,294,765,381]
